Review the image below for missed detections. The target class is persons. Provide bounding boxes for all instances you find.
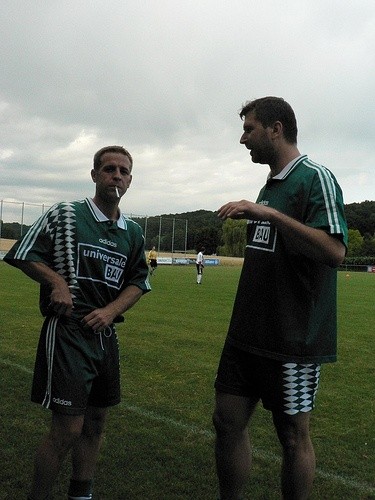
[2,146,151,500]
[212,96,349,500]
[196,247,206,284]
[148,246,157,276]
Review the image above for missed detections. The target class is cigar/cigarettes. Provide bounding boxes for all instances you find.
[114,186,120,198]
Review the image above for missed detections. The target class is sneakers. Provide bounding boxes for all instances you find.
[196,282,201,284]
[149,271,153,276]
